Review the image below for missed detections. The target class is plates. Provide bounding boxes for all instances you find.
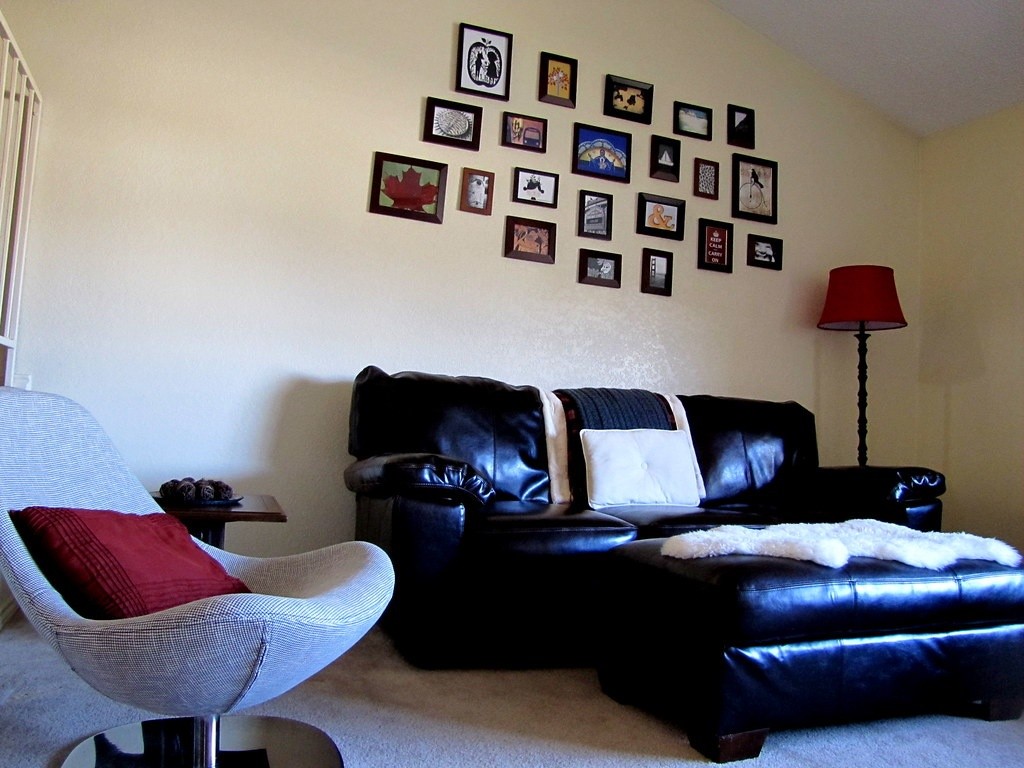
[148,490,245,505]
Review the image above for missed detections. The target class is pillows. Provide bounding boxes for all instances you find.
[581,429,700,509]
[21,506,253,619]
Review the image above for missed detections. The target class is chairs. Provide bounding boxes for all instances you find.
[0,383,396,768]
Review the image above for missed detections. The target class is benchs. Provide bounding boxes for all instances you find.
[602,519,1024,764]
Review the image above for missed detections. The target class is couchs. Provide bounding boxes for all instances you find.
[344,366,948,666]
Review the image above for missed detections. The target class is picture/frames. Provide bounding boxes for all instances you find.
[604,74,655,125]
[578,190,613,240]
[673,100,713,142]
[731,153,778,224]
[696,218,734,274]
[456,22,514,102]
[504,215,557,264]
[635,192,686,242]
[513,167,559,208]
[640,247,673,297]
[649,134,681,183]
[539,51,579,109]
[423,96,483,152]
[369,151,449,224]
[578,248,622,289]
[747,233,783,270]
[460,168,495,216]
[571,122,633,184]
[726,103,755,149]
[501,111,548,154]
[693,157,720,200]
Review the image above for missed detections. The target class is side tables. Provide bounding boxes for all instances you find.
[149,490,288,550]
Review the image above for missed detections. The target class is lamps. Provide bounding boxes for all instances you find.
[816,264,909,466]
